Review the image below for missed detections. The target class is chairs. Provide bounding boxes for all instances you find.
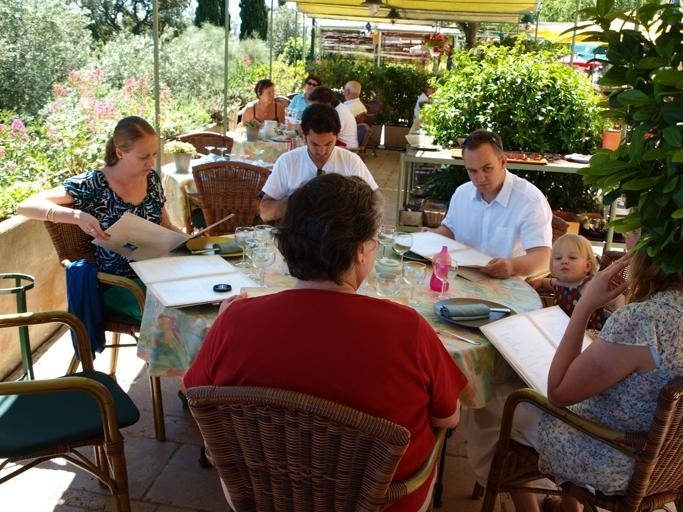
[152,72,374,239]
[183,385,454,511]
[594,246,630,300]
[0,271,146,512]
[532,213,572,306]
[45,206,167,446]
[475,371,681,512]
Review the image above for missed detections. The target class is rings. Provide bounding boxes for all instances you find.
[88,228,95,233]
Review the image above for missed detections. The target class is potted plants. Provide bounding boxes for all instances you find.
[298,60,383,150]
[373,61,430,149]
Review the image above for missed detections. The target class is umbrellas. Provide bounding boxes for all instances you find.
[531,12,681,78]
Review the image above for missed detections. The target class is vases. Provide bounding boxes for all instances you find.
[427,40,441,48]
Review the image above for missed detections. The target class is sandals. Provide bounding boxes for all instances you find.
[542,493,564,512]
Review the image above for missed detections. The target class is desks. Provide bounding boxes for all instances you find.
[396,146,628,259]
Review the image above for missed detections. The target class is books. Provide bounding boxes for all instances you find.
[476,303,595,402]
[126,252,266,309]
[88,209,234,261]
[389,227,492,267]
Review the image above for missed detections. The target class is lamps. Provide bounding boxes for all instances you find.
[517,14,535,30]
[362,0,403,25]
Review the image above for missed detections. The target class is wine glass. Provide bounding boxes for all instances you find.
[433,258,458,301]
[233,224,429,307]
[202,145,264,166]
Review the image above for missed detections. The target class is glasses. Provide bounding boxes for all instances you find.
[461,134,503,150]
[306,81,319,87]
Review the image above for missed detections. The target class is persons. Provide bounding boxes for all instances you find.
[286,73,319,119]
[507,227,682,512]
[256,102,385,226]
[416,129,553,280]
[529,232,625,331]
[241,78,283,124]
[173,169,464,511]
[15,114,197,327]
[340,80,365,115]
[306,85,360,154]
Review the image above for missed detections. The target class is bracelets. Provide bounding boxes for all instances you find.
[549,278,553,287]
[45,204,63,224]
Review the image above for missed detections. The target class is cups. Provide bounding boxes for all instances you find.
[263,119,272,138]
[280,109,300,139]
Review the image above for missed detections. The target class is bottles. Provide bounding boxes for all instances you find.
[429,245,452,292]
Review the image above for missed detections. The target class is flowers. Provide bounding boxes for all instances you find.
[418,20,445,56]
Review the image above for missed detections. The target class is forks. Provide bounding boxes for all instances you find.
[432,326,480,346]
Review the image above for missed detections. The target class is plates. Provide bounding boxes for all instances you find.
[185,236,243,257]
[410,143,438,149]
[564,154,590,163]
[432,296,517,329]
[271,135,291,142]
